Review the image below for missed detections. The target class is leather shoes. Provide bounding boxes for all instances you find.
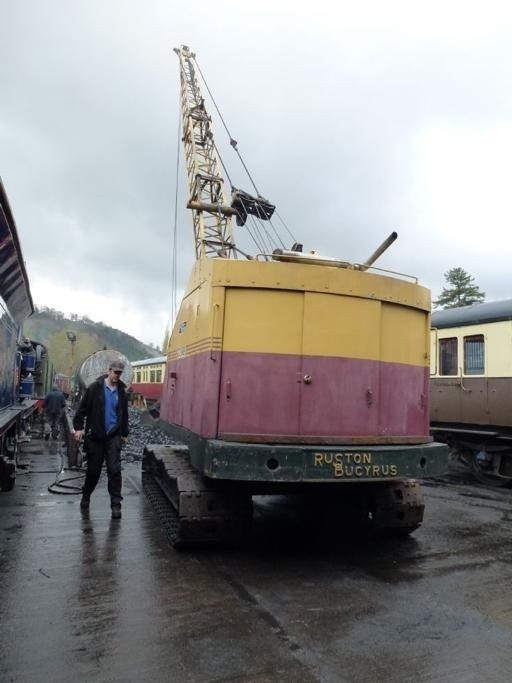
[111,506,122,518]
[79,494,90,508]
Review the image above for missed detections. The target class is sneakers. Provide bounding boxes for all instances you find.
[52,433,57,439]
[45,432,49,440]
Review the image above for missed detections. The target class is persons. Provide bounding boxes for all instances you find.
[72,359,129,519]
[39,383,66,440]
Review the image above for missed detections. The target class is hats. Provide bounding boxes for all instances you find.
[109,361,125,372]
[53,384,58,389]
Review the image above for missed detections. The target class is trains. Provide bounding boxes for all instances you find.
[130,294,512,485]
[0,175,57,468]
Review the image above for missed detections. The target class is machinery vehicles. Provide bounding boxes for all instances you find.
[137,44,454,555]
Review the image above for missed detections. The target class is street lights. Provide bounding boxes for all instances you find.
[65,330,77,378]
